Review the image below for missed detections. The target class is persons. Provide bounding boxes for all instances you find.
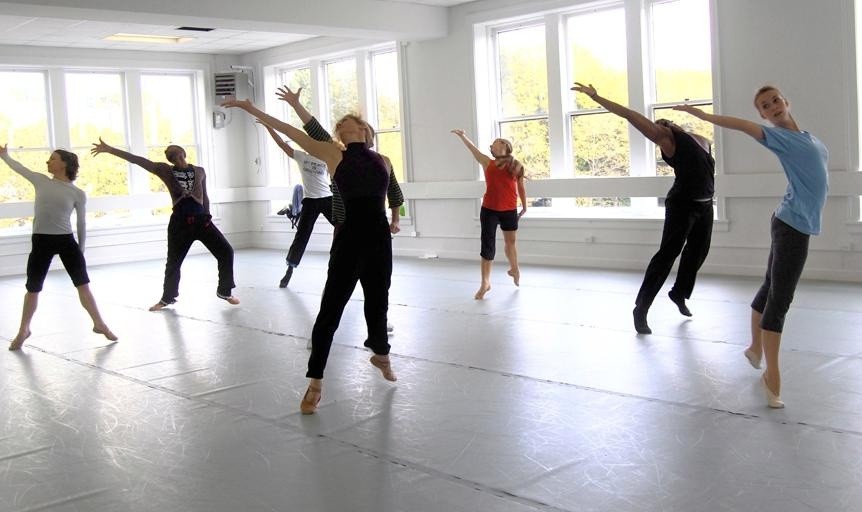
[570,82,715,335]
[0,143,119,351]
[275,85,404,331]
[451,128,526,300]
[91,136,240,311]
[673,86,829,409]
[255,117,333,288]
[219,99,397,416]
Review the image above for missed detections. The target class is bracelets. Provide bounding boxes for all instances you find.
[591,94,598,98]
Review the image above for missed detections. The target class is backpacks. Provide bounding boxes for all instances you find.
[291,183,303,217]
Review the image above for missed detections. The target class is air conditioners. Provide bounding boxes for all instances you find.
[211,72,249,107]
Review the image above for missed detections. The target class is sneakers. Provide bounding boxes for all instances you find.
[300,385,322,416]
[633,308,652,334]
[667,290,693,316]
[759,374,785,408]
[279,271,293,288]
[92,325,119,342]
[8,331,32,351]
[216,291,241,305]
[743,347,763,371]
[475,286,491,300]
[277,208,290,215]
[369,356,398,382]
[507,270,520,286]
[149,303,167,311]
